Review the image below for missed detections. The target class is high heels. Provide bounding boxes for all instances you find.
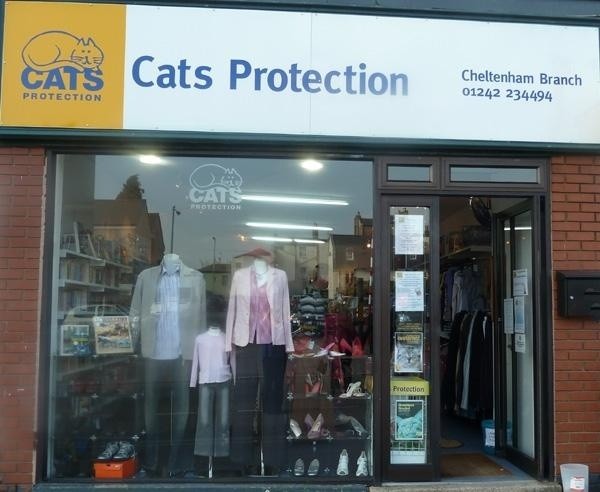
[288,337,368,442]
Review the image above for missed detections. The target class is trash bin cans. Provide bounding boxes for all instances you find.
[560,463,589,492]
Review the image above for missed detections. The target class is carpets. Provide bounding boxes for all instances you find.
[440,453,509,478]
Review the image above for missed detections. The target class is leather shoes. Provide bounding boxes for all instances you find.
[356,450,369,477]
[336,448,349,475]
[293,457,305,477]
[307,459,321,476]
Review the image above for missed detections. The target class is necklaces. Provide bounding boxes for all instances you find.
[254,267,268,277]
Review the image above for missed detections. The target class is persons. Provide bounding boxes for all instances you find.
[129,253,210,477]
[224,259,296,467]
[190,325,237,458]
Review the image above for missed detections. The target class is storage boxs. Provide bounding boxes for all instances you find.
[93,455,138,482]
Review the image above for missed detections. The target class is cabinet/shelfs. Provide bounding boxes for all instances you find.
[286,388,374,477]
[55,250,135,428]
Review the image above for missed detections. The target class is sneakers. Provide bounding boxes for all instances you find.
[97,440,133,459]
